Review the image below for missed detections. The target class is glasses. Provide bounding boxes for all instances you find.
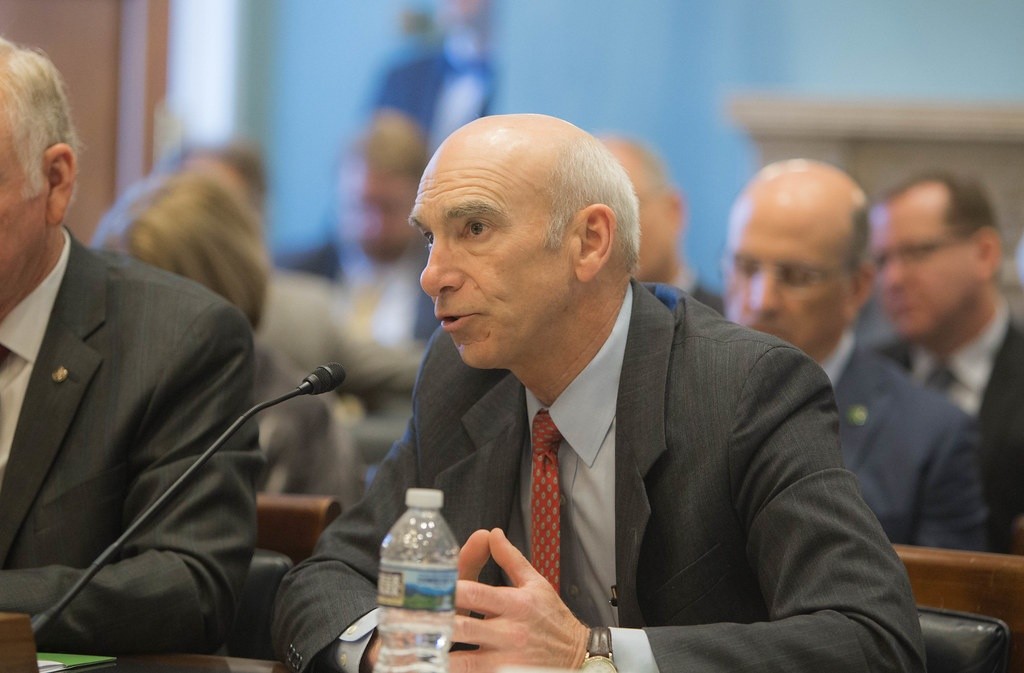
[873,239,962,268]
[730,252,860,286]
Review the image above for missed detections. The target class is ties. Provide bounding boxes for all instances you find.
[529,409,562,600]
[926,367,954,394]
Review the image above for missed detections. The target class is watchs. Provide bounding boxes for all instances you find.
[581,627,618,673]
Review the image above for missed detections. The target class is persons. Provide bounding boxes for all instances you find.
[272,113,926,672]
[276,105,449,344]
[715,159,993,552]
[89,154,328,493]
[0,36,268,647]
[866,170,1024,553]
[186,137,431,451]
[588,133,726,320]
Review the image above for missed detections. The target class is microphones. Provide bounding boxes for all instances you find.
[34,361,348,638]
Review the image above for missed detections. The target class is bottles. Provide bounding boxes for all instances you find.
[376,486,459,673]
